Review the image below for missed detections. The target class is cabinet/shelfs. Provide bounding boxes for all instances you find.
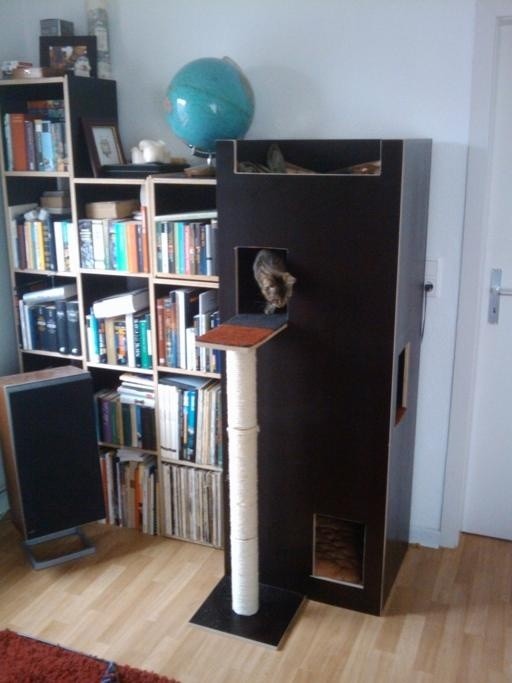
[0,76,228,549]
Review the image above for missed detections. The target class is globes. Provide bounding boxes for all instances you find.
[162,55,256,177]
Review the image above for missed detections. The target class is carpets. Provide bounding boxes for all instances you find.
[0,627,182,681]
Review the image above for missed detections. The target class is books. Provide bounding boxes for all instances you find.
[3,99,68,172]
[99,448,161,536]
[93,372,158,452]
[8,190,76,273]
[160,461,222,548]
[78,198,150,274]
[157,376,223,468]
[154,209,220,277]
[85,288,152,370]
[156,287,222,374]
[14,278,83,355]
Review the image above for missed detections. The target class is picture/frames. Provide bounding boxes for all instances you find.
[79,115,126,177]
[39,34,97,77]
